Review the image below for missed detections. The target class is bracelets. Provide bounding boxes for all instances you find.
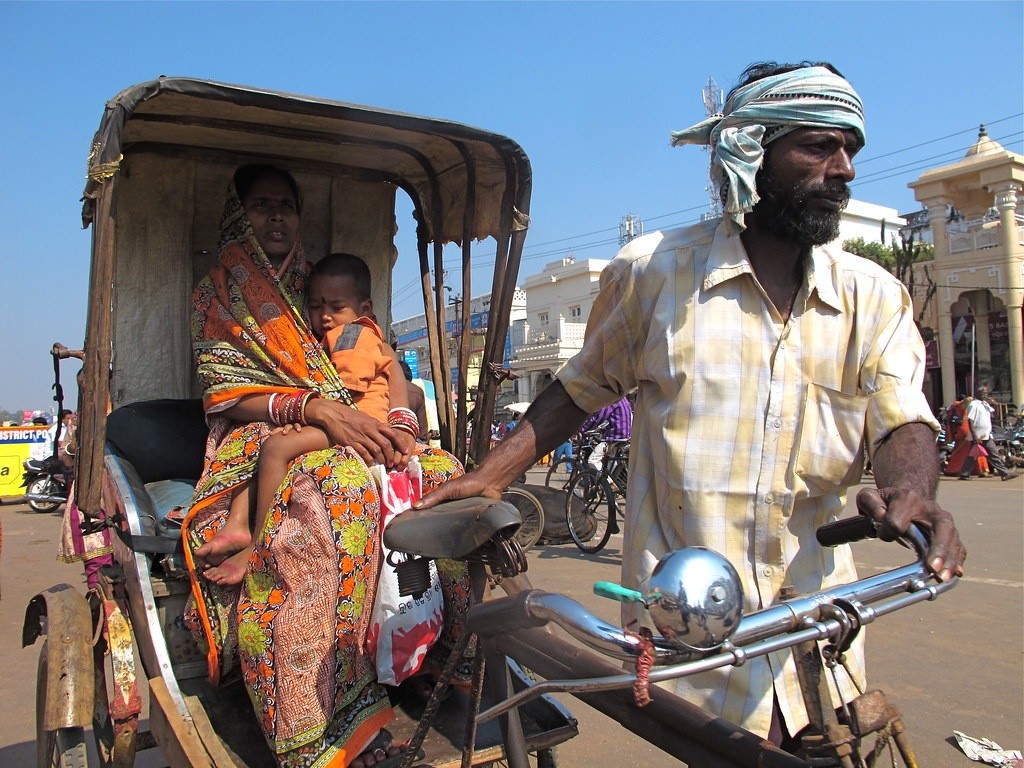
[269,391,319,426]
[65,442,76,455]
[387,407,420,441]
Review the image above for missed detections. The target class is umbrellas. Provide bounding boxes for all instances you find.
[412,377,434,401]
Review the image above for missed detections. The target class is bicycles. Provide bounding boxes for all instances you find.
[546,428,627,520]
[565,433,631,554]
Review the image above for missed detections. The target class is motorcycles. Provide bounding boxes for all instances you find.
[936,402,1024,478]
[18,455,76,514]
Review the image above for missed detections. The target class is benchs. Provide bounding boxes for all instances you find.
[102,398,212,682]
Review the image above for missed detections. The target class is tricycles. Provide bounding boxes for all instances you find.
[19,71,960,768]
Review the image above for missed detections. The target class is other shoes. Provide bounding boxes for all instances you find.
[979,473,993,477]
[958,476,968,480]
[566,471,571,473]
[600,483,619,493]
[1001,473,1018,480]
[553,469,556,473]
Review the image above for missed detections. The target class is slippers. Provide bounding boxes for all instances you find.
[414,677,456,710]
[348,728,426,768]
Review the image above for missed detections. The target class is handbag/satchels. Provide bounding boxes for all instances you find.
[969,444,989,457]
[366,456,445,685]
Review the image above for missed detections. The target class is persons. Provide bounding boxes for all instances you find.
[33,368,112,656]
[466,411,573,473]
[195,254,392,586]
[165,161,476,768]
[412,59,967,748]
[945,387,1024,481]
[398,361,439,445]
[571,396,634,499]
